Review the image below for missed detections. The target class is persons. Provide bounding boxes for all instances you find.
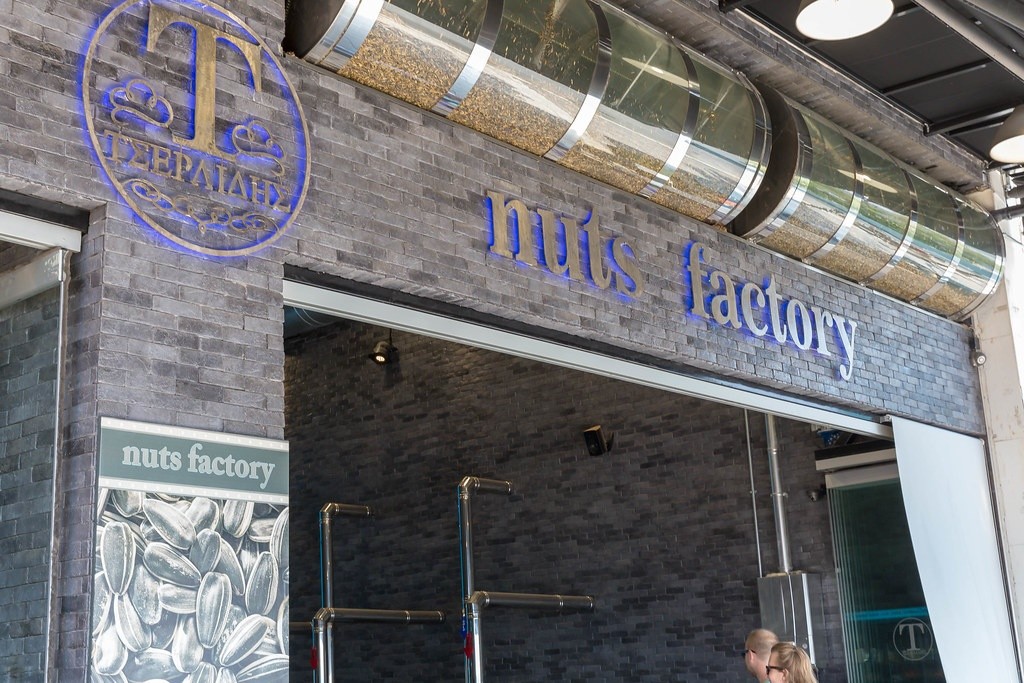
[742,628,778,683]
[766,641,818,683]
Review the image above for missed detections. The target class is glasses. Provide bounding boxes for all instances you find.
[741,650,756,657]
[766,666,790,675]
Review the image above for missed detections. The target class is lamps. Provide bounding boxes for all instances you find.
[794,0,894,41]
[368,340,396,365]
[582,424,609,457]
[988,103,1024,164]
